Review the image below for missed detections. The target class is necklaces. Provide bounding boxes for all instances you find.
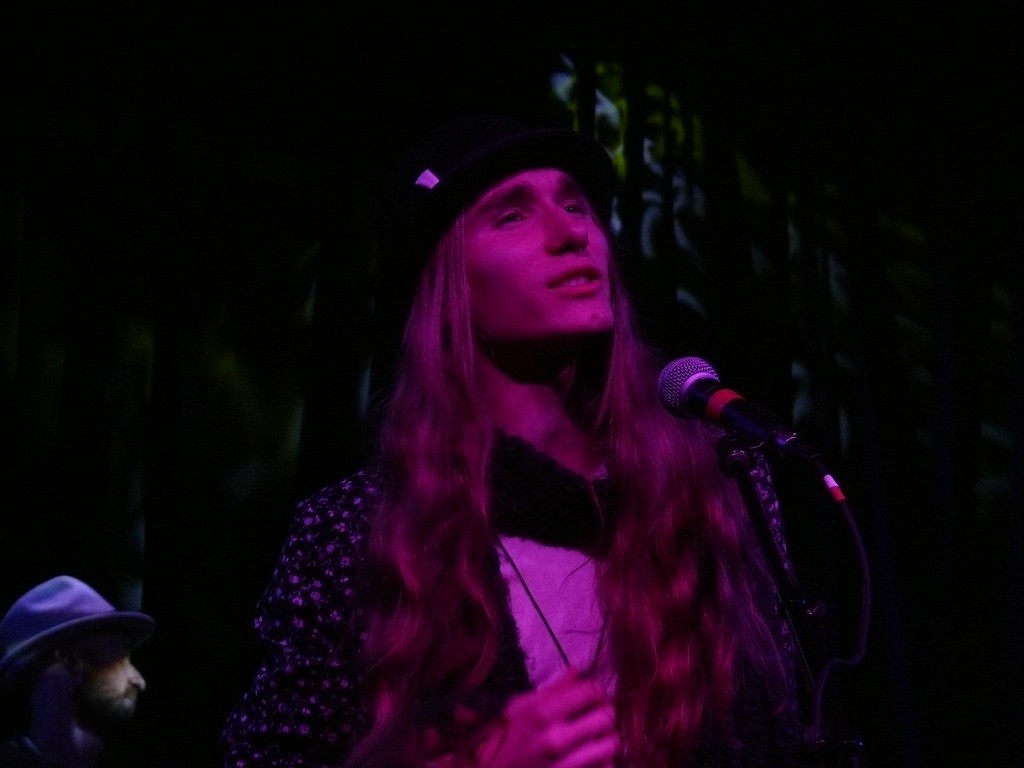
[497,534,606,679]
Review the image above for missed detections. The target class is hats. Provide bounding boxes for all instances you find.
[0,575,156,693]
[379,69,621,266]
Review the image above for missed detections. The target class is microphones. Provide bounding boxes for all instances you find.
[657,356,819,467]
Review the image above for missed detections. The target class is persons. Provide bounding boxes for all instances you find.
[218,111,803,767]
[0,576,156,768]
